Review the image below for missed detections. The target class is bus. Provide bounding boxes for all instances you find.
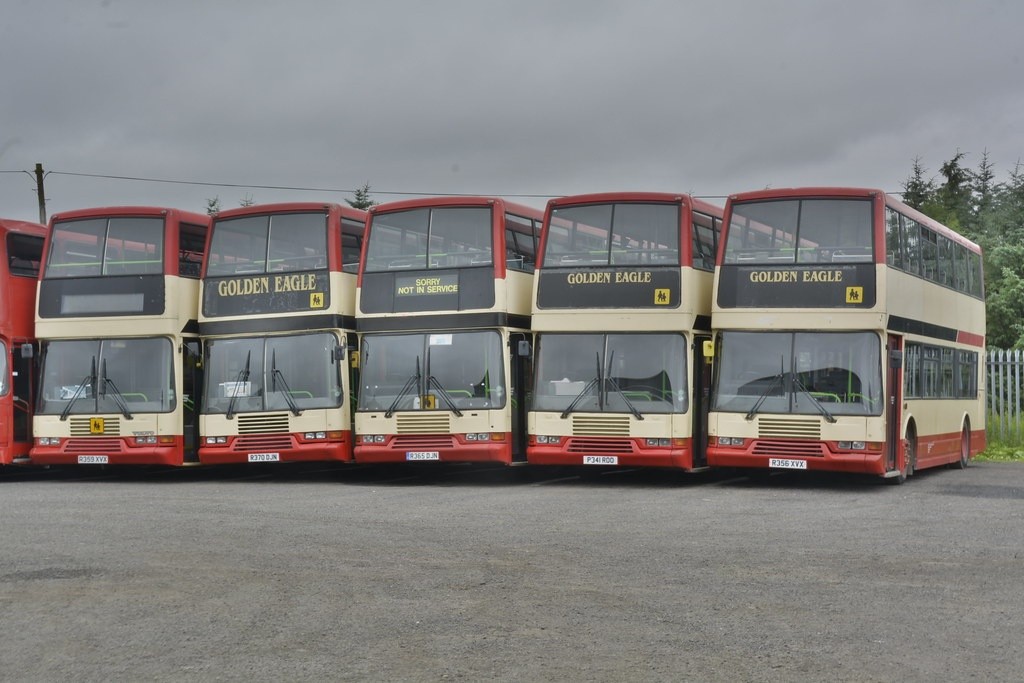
[703,186,993,478]
[198,203,486,464]
[0,221,247,464]
[523,189,825,469]
[30,205,320,463]
[351,195,668,460]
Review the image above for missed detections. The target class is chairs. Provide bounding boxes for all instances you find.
[389,252,618,270]
[736,247,966,291]
[232,262,360,275]
